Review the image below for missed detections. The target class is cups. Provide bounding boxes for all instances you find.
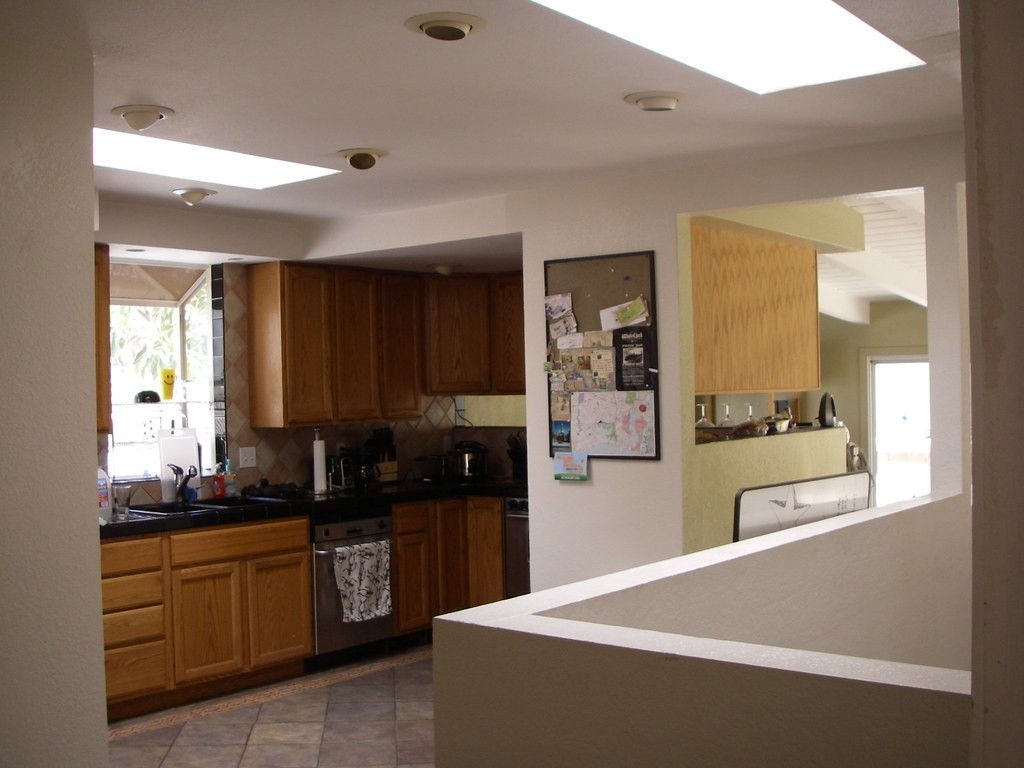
[112,484,131,518]
[161,369,175,400]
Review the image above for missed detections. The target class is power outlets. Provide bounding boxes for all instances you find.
[239,447,256,468]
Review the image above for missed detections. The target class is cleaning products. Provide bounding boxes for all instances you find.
[212,462,224,500]
[98,469,111,521]
[224,459,238,498]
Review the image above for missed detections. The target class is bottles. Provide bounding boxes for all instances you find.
[696,400,770,444]
[98,466,110,526]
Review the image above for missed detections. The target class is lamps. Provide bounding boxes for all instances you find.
[623,90,688,112]
[111,104,176,133]
[334,148,388,170]
[403,11,486,41]
[171,187,219,207]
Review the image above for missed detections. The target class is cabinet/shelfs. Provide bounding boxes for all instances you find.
[418,270,524,397]
[465,496,506,607]
[434,497,469,616]
[167,514,316,708]
[245,264,421,429]
[100,531,172,725]
[112,401,203,446]
[391,501,434,654]
[689,215,822,396]
[94,242,113,434]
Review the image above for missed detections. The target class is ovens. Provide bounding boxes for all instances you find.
[312,516,396,655]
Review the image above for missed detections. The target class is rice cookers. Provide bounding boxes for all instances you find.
[440,441,488,490]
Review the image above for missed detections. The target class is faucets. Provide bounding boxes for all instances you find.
[168,463,197,501]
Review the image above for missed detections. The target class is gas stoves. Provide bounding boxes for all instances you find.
[240,480,393,521]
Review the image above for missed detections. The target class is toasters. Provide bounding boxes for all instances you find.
[324,453,358,490]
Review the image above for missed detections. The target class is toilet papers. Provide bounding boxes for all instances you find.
[313,440,327,492]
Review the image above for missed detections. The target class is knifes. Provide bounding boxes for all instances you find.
[366,428,397,466]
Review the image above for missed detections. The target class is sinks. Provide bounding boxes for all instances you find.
[130,502,231,516]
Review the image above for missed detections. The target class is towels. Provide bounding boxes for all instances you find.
[332,538,392,624]
